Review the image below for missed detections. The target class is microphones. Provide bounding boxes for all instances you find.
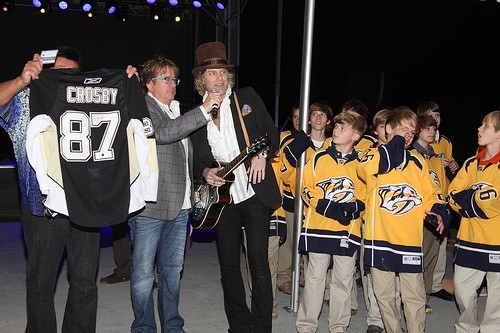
[210,88,220,118]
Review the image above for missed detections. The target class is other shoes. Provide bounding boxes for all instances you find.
[352,308,357,315]
[271,307,277,317]
[100,272,126,285]
[300,268,305,286]
[431,289,456,301]
[366,325,383,333]
[425,303,433,313]
[278,281,292,294]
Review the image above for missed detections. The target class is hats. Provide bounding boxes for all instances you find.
[192,42,236,79]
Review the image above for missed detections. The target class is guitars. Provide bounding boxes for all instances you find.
[191,133,272,230]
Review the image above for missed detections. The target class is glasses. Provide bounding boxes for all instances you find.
[150,76,180,86]
[309,111,326,118]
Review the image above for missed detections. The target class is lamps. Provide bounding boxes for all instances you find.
[81,11,96,21]
[216,0,228,11]
[115,9,129,25]
[40,0,51,14]
[167,0,181,9]
[31,0,46,9]
[57,0,71,11]
[169,9,185,25]
[148,6,164,23]
[80,0,98,14]
[104,0,119,16]
[191,0,205,9]
[2,0,15,14]
[146,0,161,7]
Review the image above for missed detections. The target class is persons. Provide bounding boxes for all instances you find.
[192,43,284,333]
[128,54,220,333]
[448,111,500,333]
[0,48,140,333]
[100,213,193,284]
[241,100,455,333]
